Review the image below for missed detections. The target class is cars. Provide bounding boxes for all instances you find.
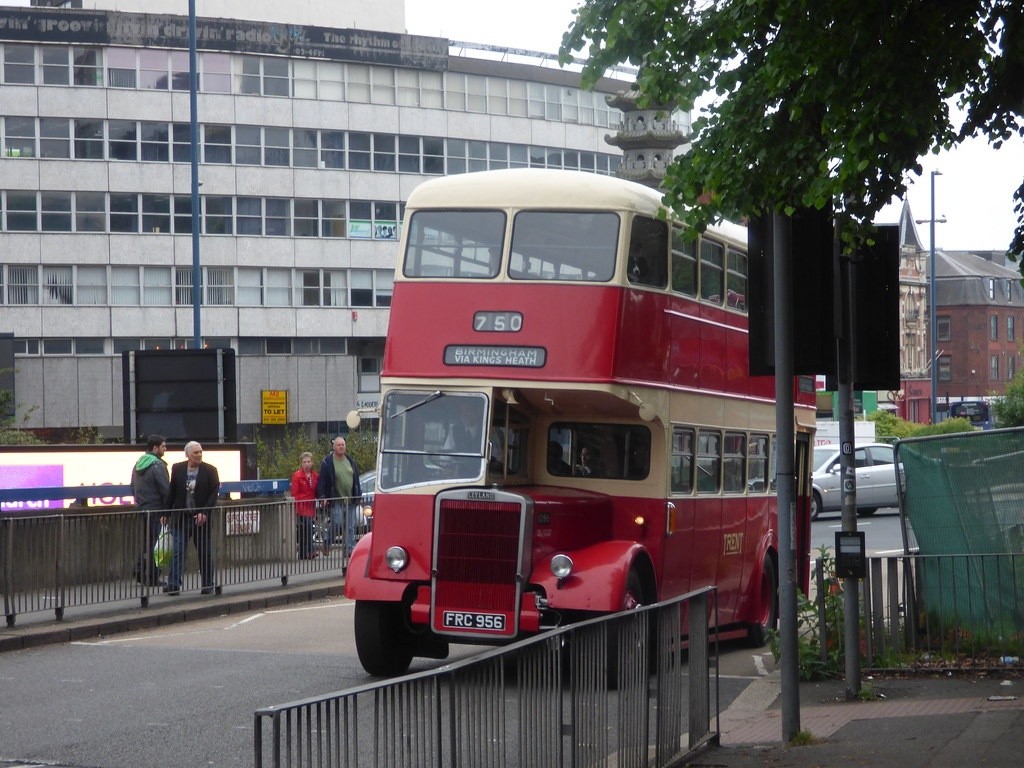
[312,468,391,545]
[811,441,905,523]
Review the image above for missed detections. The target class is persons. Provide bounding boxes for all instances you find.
[548,441,572,475]
[160,441,220,596]
[441,402,505,475]
[290,452,326,559]
[131,435,170,586]
[316,437,361,558]
[572,447,606,477]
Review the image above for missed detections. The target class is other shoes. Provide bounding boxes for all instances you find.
[299,552,319,559]
[343,548,351,558]
[135,570,166,587]
[321,544,331,557]
[202,583,214,594]
[163,585,181,596]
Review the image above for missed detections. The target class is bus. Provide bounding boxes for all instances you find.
[342,168,819,681]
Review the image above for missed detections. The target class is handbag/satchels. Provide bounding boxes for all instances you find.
[154,523,175,569]
[355,504,368,527]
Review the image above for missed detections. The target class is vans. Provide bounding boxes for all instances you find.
[947,400,995,432]
